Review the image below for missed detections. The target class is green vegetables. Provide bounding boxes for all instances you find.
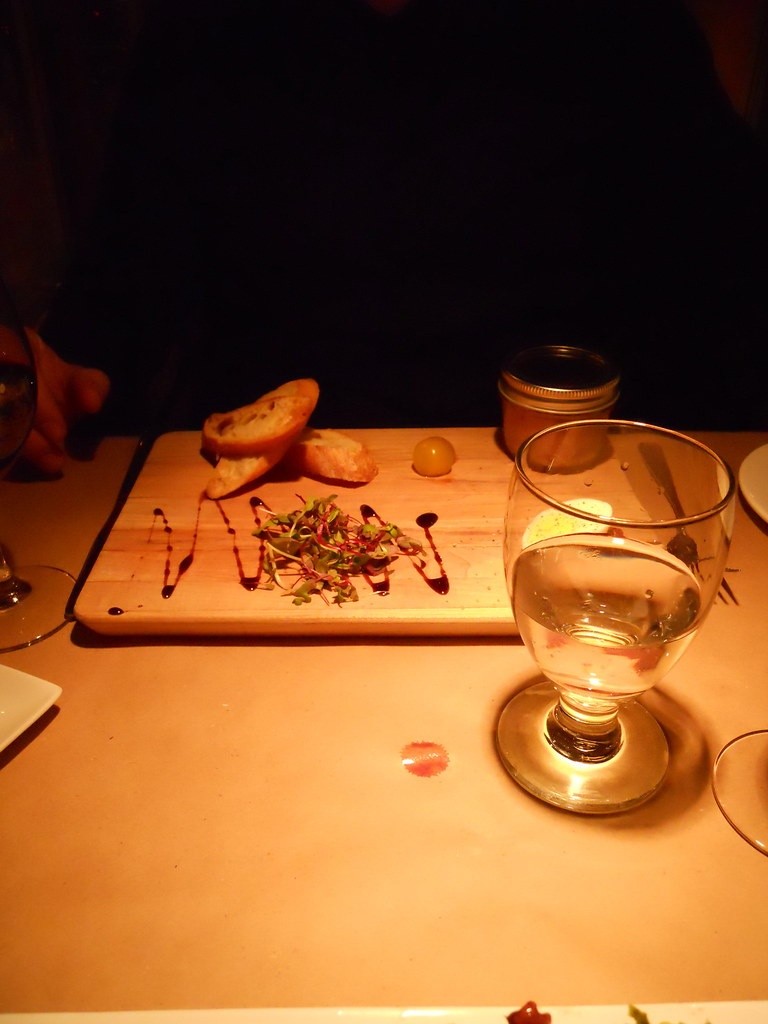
[248,494,398,607]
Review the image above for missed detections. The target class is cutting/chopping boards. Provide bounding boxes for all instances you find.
[75,426,665,634]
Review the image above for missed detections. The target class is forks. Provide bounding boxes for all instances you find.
[639,442,705,582]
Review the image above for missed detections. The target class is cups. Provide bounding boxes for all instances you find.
[499,340,621,469]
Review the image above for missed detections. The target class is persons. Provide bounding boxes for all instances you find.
[0,0,768,474]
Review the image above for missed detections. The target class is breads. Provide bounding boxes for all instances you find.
[201,377,378,500]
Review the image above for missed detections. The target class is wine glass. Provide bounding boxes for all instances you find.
[497,419,733,815]
[0,290,77,652]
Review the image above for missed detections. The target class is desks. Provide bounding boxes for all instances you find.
[0,436,768,1017]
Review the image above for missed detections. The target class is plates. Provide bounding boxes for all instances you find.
[0,664,63,751]
[738,444,768,523]
